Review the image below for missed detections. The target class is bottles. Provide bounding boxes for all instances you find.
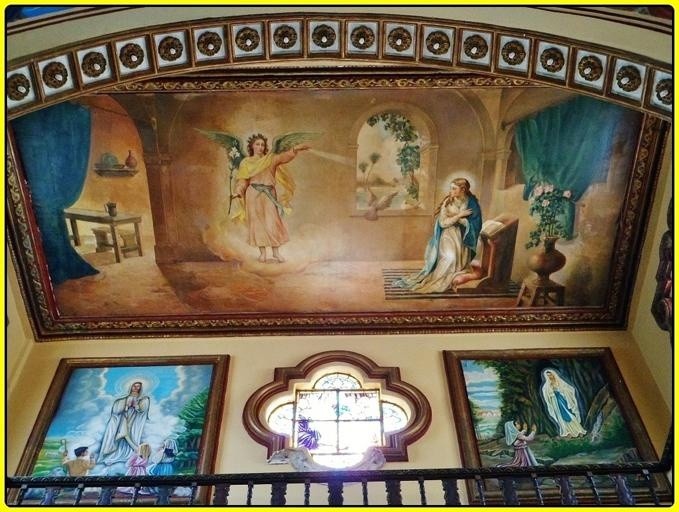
[125,150,138,168]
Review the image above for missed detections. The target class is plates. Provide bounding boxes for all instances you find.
[112,164,124,169]
[94,163,105,170]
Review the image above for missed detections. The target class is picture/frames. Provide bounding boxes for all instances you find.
[442,347,673,505]
[8,67,672,344]
[6,355,231,505]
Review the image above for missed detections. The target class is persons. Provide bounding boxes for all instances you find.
[117,443,152,495]
[61,446,95,477]
[391,178,482,293]
[96,381,150,466]
[149,439,178,494]
[235,134,312,263]
[495,420,542,467]
[541,369,587,438]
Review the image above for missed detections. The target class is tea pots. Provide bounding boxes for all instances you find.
[104,202,117,216]
[102,151,117,169]
